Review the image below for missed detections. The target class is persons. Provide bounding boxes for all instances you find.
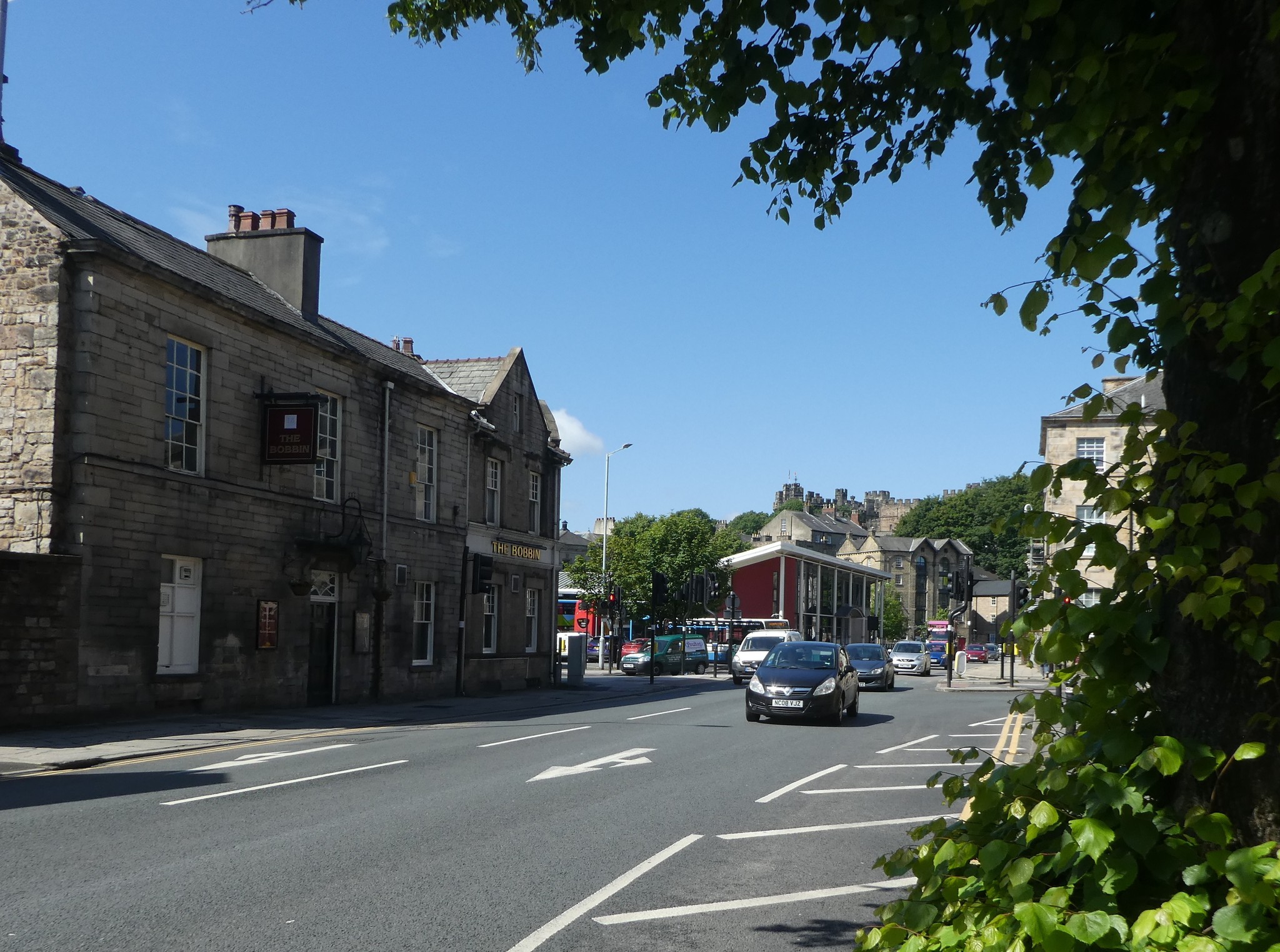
[927,634,935,640]
[1040,664,1049,679]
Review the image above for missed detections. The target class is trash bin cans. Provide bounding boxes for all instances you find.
[1041,663,1054,677]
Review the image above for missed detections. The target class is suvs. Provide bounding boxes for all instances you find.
[586,635,631,663]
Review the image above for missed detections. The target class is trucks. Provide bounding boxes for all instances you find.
[619,634,709,676]
[557,632,594,658]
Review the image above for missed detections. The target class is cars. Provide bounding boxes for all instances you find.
[745,640,860,726]
[887,641,931,676]
[844,643,895,693]
[925,642,947,670]
[621,638,650,658]
[985,643,1003,661]
[965,644,989,664]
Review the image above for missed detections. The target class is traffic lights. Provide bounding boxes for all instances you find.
[673,591,682,601]
[1015,587,1029,609]
[706,572,720,600]
[651,567,670,606]
[947,571,960,601]
[606,592,616,609]
[613,586,624,611]
[596,598,608,615]
[681,582,689,599]
[1054,586,1071,604]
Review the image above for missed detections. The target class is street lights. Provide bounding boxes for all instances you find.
[601,443,634,590]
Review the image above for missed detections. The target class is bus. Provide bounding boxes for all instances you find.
[669,618,790,666]
[556,588,610,638]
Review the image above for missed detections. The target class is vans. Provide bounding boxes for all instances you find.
[731,628,803,685]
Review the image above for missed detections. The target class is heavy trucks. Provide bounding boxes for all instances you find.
[923,620,958,661]
